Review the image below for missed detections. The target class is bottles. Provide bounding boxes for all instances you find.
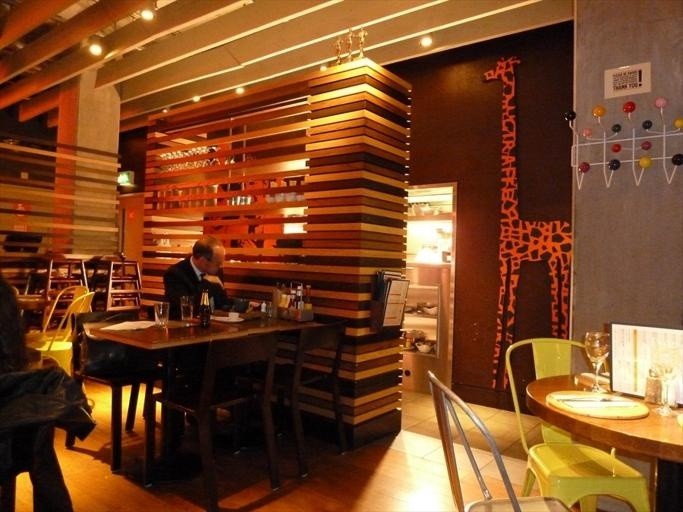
[198,288,211,330]
[287,284,313,311]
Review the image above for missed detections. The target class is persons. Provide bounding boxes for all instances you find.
[1,274,43,376]
[164,237,263,319]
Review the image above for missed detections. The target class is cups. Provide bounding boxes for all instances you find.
[179,295,195,322]
[153,301,170,328]
[229,312,239,320]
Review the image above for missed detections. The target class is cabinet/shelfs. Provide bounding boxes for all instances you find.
[400,183,453,396]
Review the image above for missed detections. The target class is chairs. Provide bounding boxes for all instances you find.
[506,337,652,511]
[271,321,346,479]
[66,305,177,475]
[145,330,282,511]
[423,369,564,511]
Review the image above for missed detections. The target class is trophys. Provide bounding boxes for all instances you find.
[334,25,368,65]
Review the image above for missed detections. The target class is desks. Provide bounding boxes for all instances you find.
[90,309,346,487]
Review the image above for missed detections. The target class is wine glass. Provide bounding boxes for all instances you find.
[583,329,611,396]
